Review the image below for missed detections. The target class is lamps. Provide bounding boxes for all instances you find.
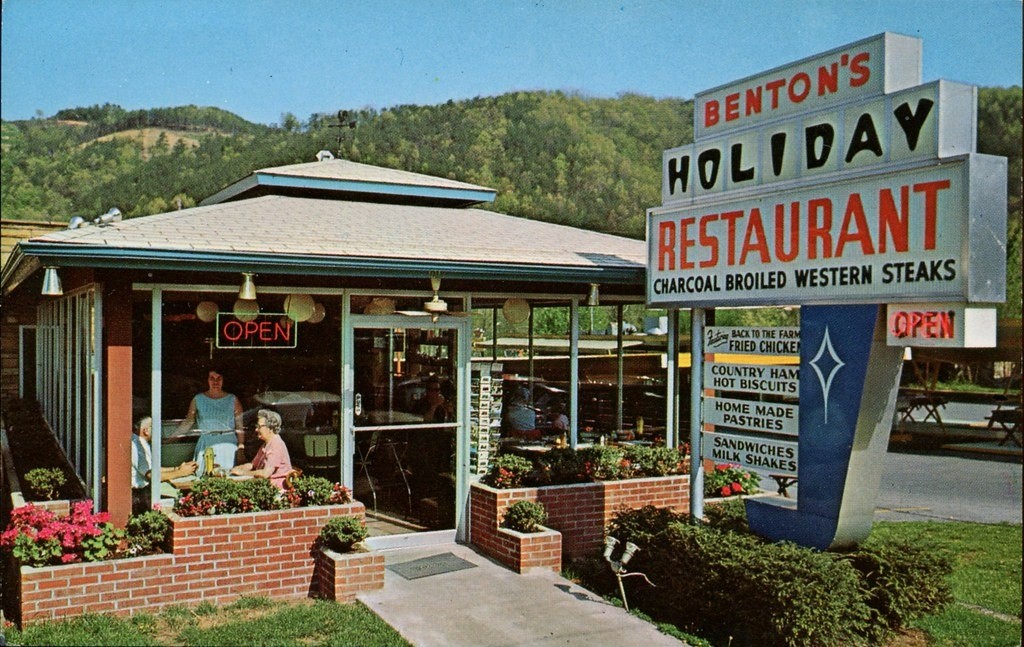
[94,207,123,227]
[239,271,258,300]
[617,541,642,566]
[67,217,84,232]
[588,281,601,307]
[40,266,63,295]
[364,296,397,316]
[234,299,261,323]
[602,535,620,564]
[285,294,325,324]
[196,300,219,322]
[503,298,532,324]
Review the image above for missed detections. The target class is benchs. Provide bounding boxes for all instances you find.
[769,472,799,498]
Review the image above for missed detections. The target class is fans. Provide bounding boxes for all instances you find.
[390,277,482,324]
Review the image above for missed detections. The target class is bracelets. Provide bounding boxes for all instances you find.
[174,465,177,470]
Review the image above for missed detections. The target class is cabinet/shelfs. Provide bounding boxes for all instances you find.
[404,328,455,397]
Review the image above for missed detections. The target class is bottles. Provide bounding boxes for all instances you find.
[560,431,567,447]
[637,416,643,434]
[203,448,214,473]
[599,434,605,446]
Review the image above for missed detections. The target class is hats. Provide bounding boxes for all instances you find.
[513,388,530,400]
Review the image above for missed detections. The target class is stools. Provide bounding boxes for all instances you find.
[352,428,414,498]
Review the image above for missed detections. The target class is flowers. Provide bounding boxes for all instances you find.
[177,485,260,519]
[0,498,113,566]
[279,483,353,510]
[708,459,759,500]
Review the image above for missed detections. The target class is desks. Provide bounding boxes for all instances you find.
[363,414,425,487]
[984,415,1024,449]
[505,439,596,461]
[168,469,257,491]
[902,397,948,425]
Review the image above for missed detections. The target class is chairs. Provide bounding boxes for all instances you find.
[303,433,340,484]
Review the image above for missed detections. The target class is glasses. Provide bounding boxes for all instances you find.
[255,424,266,428]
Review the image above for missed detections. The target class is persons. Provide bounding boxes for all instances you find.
[230,409,293,495]
[409,379,570,497]
[167,359,245,477]
[130,415,198,514]
